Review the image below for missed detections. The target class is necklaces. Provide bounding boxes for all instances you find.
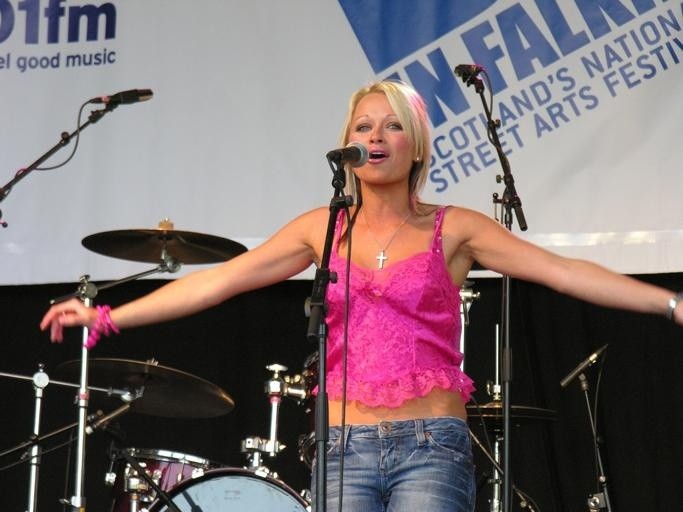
[361,207,411,269]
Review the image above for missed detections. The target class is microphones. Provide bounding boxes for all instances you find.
[454,64,484,76]
[85,404,131,436]
[559,344,608,388]
[327,140,370,168]
[90,88,154,104]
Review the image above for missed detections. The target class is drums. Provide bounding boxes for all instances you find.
[145,467,308,512]
[99,447,209,512]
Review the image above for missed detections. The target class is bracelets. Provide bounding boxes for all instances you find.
[665,291,683,320]
[84,303,120,350]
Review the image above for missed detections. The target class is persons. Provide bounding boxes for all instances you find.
[36,79,683,512]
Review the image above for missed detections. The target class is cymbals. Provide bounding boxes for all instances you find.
[54,357,235,418]
[82,227,248,264]
[466,402,558,421]
